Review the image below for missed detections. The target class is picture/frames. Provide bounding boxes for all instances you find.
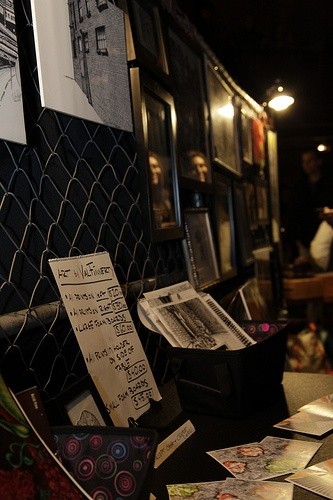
[238,109,270,232]
[202,53,240,177]
[132,0,169,75]
[138,76,186,243]
[226,179,253,269]
[178,206,222,291]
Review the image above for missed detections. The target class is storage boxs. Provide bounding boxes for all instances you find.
[165,318,290,416]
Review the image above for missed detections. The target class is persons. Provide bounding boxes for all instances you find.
[289,149,333,272]
[184,152,208,183]
[148,152,172,223]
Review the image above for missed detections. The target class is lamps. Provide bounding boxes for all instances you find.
[266,77,295,112]
